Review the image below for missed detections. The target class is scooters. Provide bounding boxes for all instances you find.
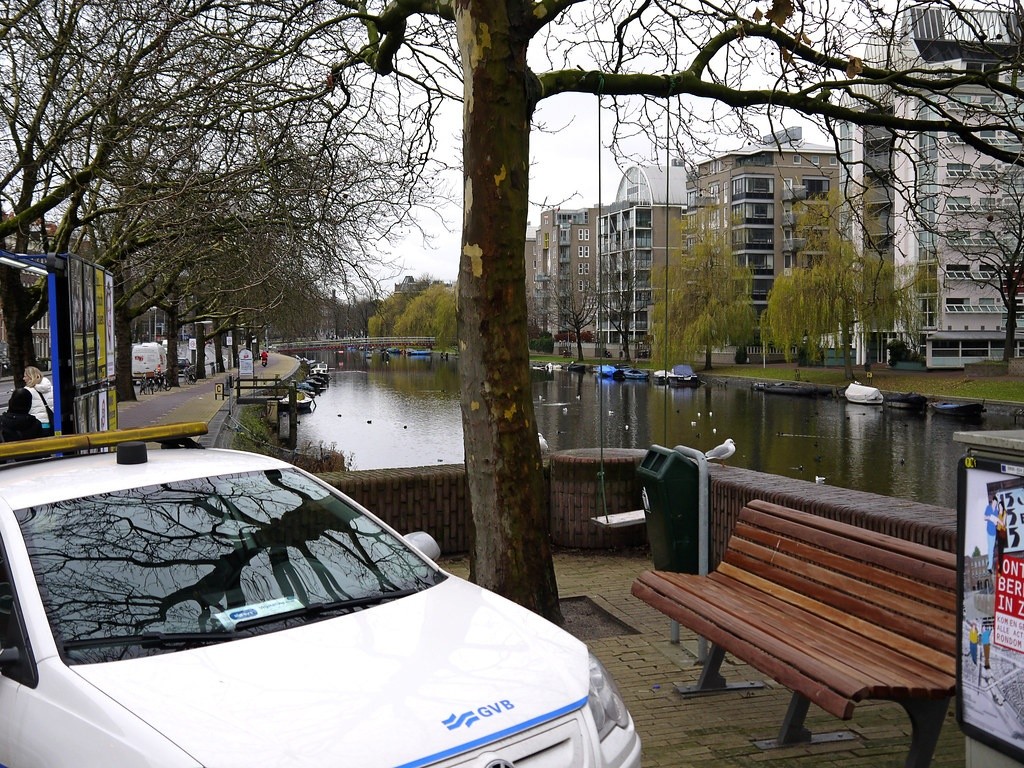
[260,355,268,367]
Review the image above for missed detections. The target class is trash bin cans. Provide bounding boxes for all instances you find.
[634,445,713,576]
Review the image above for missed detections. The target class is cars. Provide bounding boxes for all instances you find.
[176,357,191,376]
[0,421,643,768]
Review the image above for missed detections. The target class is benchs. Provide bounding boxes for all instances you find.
[628,498,958,767]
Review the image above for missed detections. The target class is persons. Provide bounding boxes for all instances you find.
[21,366,54,436]
[261,351,269,364]
[0,388,44,445]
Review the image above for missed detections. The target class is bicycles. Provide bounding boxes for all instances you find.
[136,372,154,395]
[151,372,172,392]
[184,364,196,384]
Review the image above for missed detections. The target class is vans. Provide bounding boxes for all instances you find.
[132,342,167,385]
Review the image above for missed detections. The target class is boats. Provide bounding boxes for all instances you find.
[930,401,984,416]
[544,359,698,382]
[845,383,885,404]
[885,392,928,409]
[410,351,433,356]
[278,354,330,410]
[763,382,835,396]
